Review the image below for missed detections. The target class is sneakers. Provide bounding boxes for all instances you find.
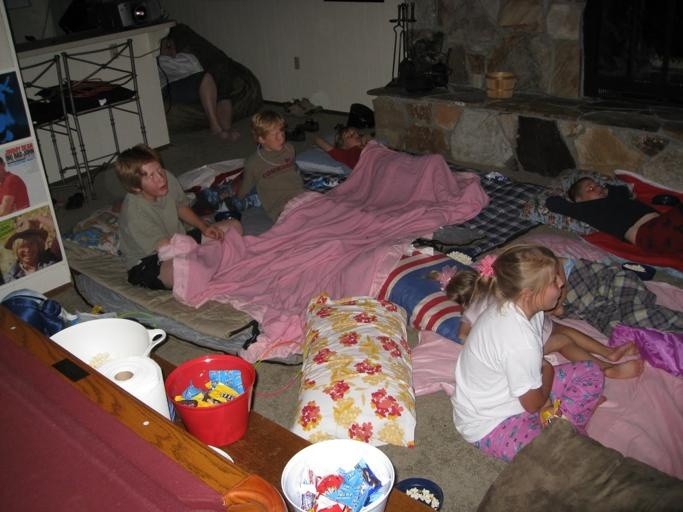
[282,98,324,119]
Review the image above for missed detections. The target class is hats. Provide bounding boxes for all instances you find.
[4,220,48,249]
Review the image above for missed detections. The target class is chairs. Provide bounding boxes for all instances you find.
[61,38,150,201]
[17,52,86,203]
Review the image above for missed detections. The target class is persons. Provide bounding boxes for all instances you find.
[451,244,605,462]
[0,76,18,143]
[2,218,59,287]
[115,144,243,290]
[159,37,242,142]
[545,176,682,253]
[219,110,308,224]
[314,122,374,169]
[445,253,683,379]
[1,157,30,216]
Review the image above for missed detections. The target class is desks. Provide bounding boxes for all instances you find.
[0,301,436,511]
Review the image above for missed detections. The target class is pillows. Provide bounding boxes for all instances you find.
[296,137,352,176]
[475,418,682,512]
[66,202,125,256]
[523,167,635,237]
[290,292,415,447]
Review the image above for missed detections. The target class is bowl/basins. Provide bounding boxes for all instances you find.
[47,317,166,375]
[649,192,680,207]
[621,261,655,282]
[212,210,242,223]
[395,476,444,511]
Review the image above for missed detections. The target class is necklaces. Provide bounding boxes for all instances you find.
[255,143,295,168]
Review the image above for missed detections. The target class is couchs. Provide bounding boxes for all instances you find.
[149,18,263,132]
